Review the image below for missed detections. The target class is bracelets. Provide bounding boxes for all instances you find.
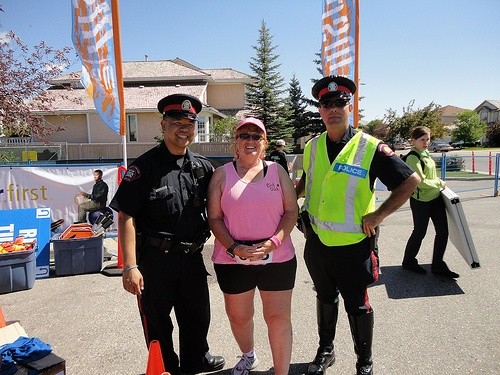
[269,235,282,249]
[226,242,240,258]
[122,265,138,273]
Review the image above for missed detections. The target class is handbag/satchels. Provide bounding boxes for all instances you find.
[400,151,425,171]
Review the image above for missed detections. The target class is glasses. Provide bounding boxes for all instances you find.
[239,133,266,140]
[320,98,350,108]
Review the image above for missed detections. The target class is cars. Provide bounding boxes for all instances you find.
[451,140,476,149]
[428,141,452,153]
[385,139,412,150]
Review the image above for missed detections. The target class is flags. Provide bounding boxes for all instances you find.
[322,0,360,129]
[71,0,126,135]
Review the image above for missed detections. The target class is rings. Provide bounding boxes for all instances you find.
[128,281,131,285]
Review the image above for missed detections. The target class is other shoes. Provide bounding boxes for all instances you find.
[308,347,335,375]
[402,256,426,274]
[356,360,373,375]
[233,353,259,375]
[432,261,459,278]
[204,355,226,371]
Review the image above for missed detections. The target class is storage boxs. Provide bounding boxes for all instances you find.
[0,322,66,375]
[51,233,104,274]
[0,243,37,293]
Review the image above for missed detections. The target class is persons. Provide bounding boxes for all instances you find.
[116,92,217,375]
[292,75,421,375]
[206,116,299,375]
[74,170,108,224]
[268,138,290,178]
[403,125,461,277]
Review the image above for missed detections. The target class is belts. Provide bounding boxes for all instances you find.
[145,236,205,252]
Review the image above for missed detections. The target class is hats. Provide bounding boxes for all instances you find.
[235,117,266,133]
[312,76,356,97]
[276,139,286,146]
[158,93,202,121]
